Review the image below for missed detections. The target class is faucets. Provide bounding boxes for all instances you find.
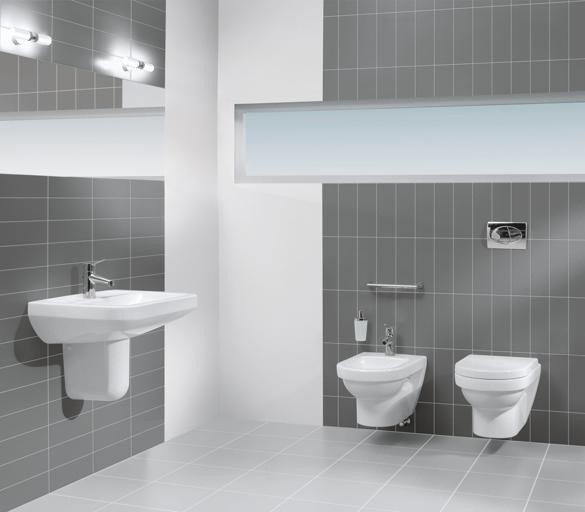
[382,323,394,355]
[83,259,114,297]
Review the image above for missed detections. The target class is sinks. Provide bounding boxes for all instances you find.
[27,289,198,345]
[336,352,427,401]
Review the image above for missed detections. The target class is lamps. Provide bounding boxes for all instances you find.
[5,25,53,52]
[122,54,154,75]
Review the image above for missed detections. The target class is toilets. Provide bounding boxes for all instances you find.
[454,354,541,438]
